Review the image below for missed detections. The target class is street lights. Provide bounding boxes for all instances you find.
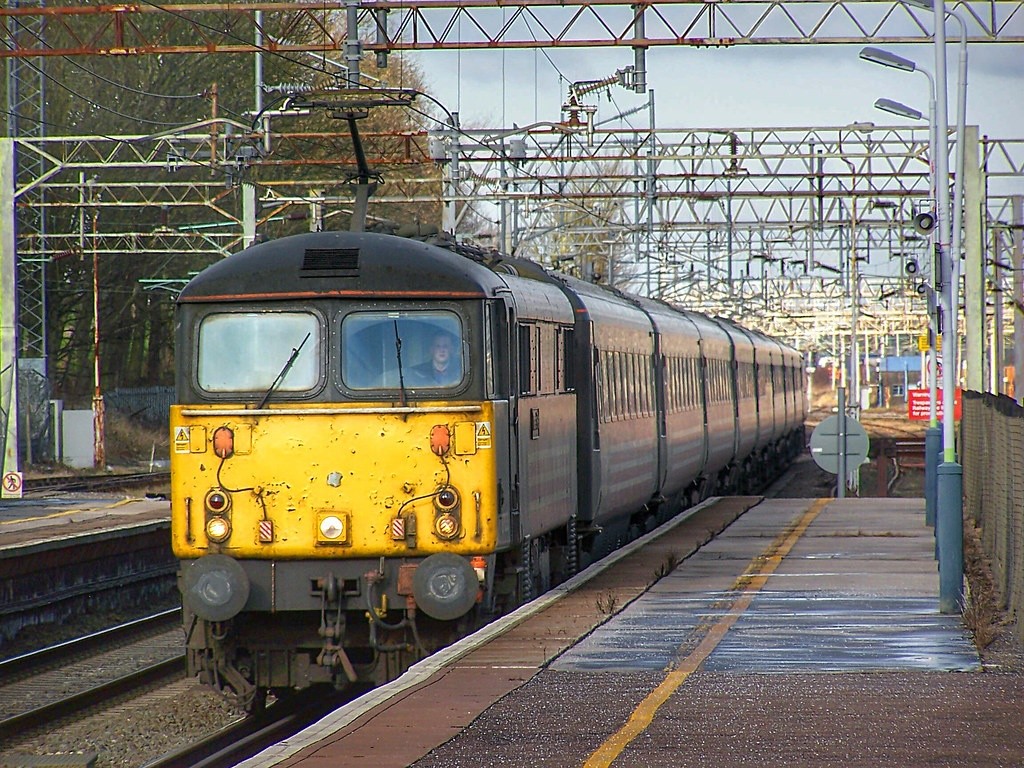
[859,45,943,528]
[873,97,958,466]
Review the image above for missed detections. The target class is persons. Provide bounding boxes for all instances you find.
[412,329,461,385]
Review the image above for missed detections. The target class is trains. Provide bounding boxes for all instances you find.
[169,227,816,716]
